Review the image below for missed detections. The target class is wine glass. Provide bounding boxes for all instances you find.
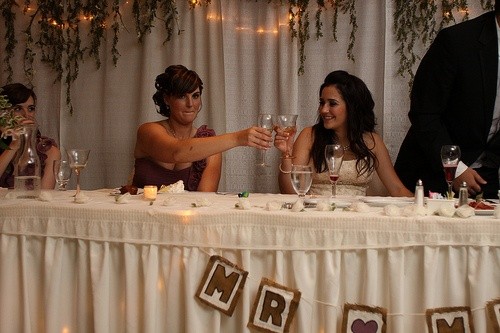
[255,114,277,166]
[277,114,299,159]
[64,148,90,197]
[52,160,73,192]
[326,144,345,199]
[291,162,313,208]
[441,145,461,199]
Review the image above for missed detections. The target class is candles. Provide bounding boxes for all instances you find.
[144,185,157,199]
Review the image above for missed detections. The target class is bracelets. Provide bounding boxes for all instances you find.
[279,163,293,174]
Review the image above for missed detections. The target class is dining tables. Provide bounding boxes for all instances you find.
[0,190,500,333]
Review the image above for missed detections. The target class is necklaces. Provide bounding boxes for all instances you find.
[334,143,351,151]
[170,124,193,139]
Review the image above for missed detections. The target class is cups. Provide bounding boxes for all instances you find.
[19,116,40,127]
[144,185,157,200]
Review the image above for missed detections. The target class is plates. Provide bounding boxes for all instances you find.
[111,187,144,197]
[160,190,500,216]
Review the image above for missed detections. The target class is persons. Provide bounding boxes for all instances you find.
[131,65,274,192]
[393,0,500,202]
[0,83,62,191]
[273,70,415,198]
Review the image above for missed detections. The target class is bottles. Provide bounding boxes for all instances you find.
[14,124,40,198]
[459,180,469,206]
[414,179,424,207]
